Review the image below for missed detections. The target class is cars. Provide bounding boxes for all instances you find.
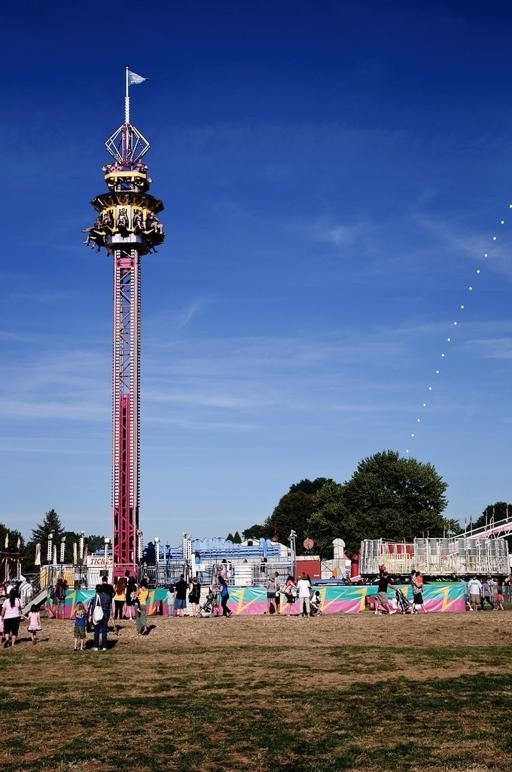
[12,573,41,594]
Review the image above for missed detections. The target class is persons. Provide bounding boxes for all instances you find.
[0,552,233,652]
[266,571,322,618]
[374,564,429,616]
[81,210,163,257]
[460,576,505,611]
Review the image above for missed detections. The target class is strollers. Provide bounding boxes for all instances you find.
[196,587,217,618]
[388,584,418,615]
[297,589,322,617]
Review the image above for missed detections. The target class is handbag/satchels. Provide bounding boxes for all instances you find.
[93,606,104,626]
